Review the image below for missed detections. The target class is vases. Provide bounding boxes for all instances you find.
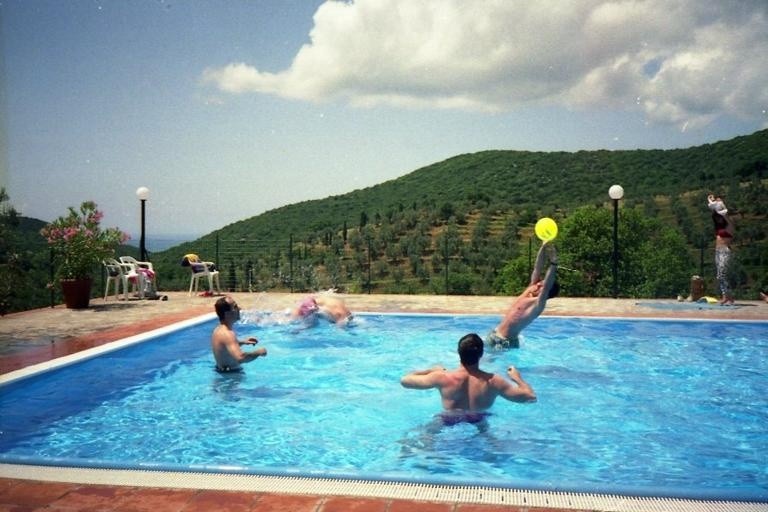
[59,278,93,309]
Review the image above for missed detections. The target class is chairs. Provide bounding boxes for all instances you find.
[100,255,156,303]
[187,254,220,295]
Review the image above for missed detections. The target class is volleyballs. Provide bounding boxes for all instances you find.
[536,217,559,241]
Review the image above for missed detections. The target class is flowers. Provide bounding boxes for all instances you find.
[40,200,130,290]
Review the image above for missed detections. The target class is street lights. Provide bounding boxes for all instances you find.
[608,184,624,298]
[135,186,150,262]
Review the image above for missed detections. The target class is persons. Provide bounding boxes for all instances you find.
[211,294,267,374]
[400,333,538,431]
[707,195,734,306]
[484,239,558,349]
[287,293,357,333]
